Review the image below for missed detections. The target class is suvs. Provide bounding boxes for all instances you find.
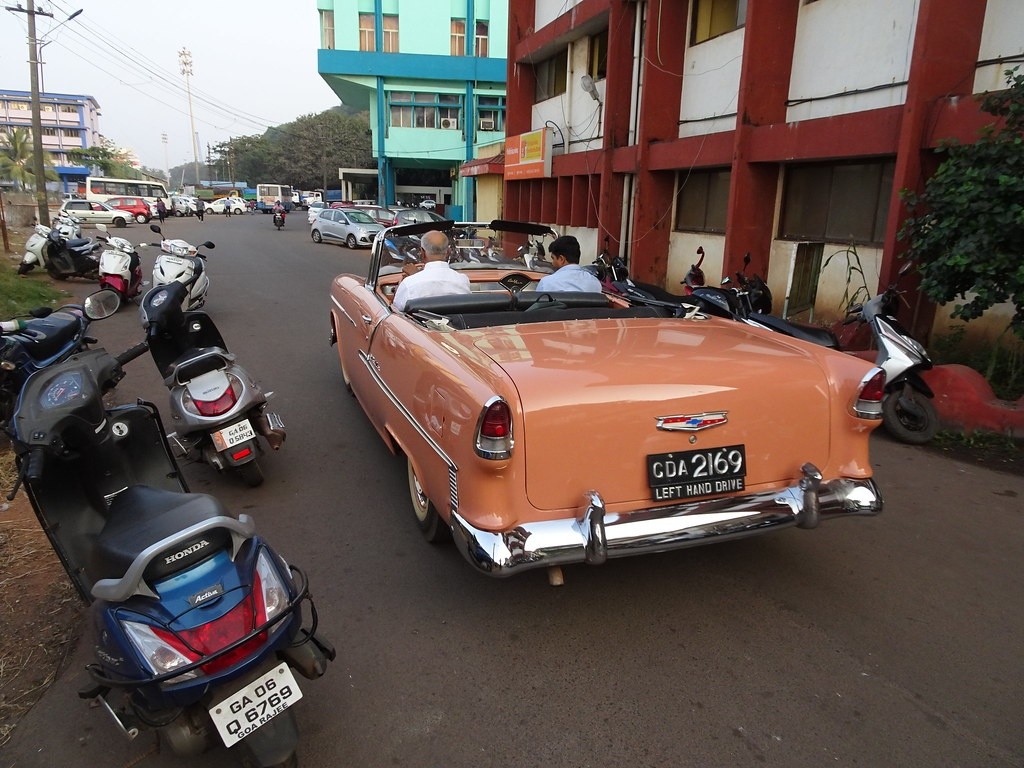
[740,259,939,446]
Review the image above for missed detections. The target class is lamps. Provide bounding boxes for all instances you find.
[581,75,602,101]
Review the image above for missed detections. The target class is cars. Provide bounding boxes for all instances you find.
[169,180,456,235]
[310,208,387,250]
[56,200,135,228]
[91,197,153,224]
[328,219,888,588]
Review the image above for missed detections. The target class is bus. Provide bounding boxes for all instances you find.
[86,176,173,217]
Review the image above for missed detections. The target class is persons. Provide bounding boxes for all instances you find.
[397,200,402,206]
[196,195,205,223]
[225,197,231,217]
[250,199,256,215]
[394,230,470,311]
[535,235,602,293]
[156,197,167,224]
[272,200,285,223]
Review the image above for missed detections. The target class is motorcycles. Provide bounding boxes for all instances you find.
[599,249,773,324]
[271,208,287,231]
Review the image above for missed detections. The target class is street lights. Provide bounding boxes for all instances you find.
[29,8,84,227]
[178,46,202,184]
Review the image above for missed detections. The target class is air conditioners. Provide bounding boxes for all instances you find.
[440,118,457,130]
[481,120,494,130]
[450,167,456,178]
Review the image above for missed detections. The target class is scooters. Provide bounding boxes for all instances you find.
[131,272,288,490]
[5,337,338,768]
[148,225,216,314]
[51,209,88,244]
[592,236,707,302]
[45,219,105,283]
[95,223,150,313]
[16,216,75,276]
[0,301,98,437]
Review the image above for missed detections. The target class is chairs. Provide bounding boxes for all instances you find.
[514,291,610,309]
[404,290,513,314]
